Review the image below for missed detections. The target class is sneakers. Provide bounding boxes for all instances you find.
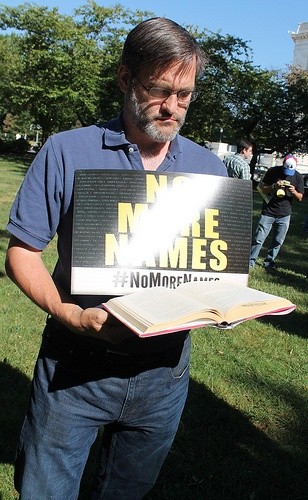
[249,260,256,270]
[265,265,280,276]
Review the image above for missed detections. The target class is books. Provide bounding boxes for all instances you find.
[101,281,296,337]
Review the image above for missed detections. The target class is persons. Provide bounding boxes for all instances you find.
[223,140,253,180]
[249,155,304,268]
[6,18,227,500]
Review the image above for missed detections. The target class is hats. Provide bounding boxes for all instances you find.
[282,156,298,176]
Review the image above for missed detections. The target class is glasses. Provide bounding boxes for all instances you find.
[133,74,199,103]
